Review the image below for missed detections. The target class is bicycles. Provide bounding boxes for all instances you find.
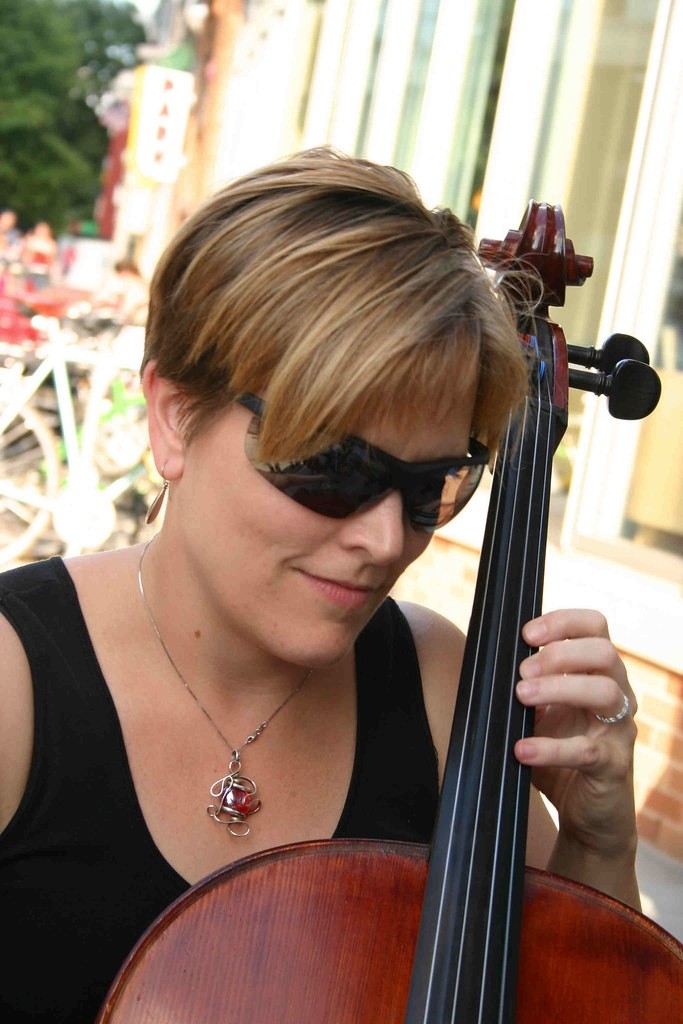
[0,314,149,558]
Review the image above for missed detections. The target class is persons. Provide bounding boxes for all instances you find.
[0,149,646,1024]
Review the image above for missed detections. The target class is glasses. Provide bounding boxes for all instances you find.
[223,388,491,534]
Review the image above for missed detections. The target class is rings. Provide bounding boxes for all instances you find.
[594,693,629,725]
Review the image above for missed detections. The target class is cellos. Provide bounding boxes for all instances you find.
[97,195,683,1023]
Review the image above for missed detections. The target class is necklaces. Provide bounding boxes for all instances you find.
[138,534,313,840]
